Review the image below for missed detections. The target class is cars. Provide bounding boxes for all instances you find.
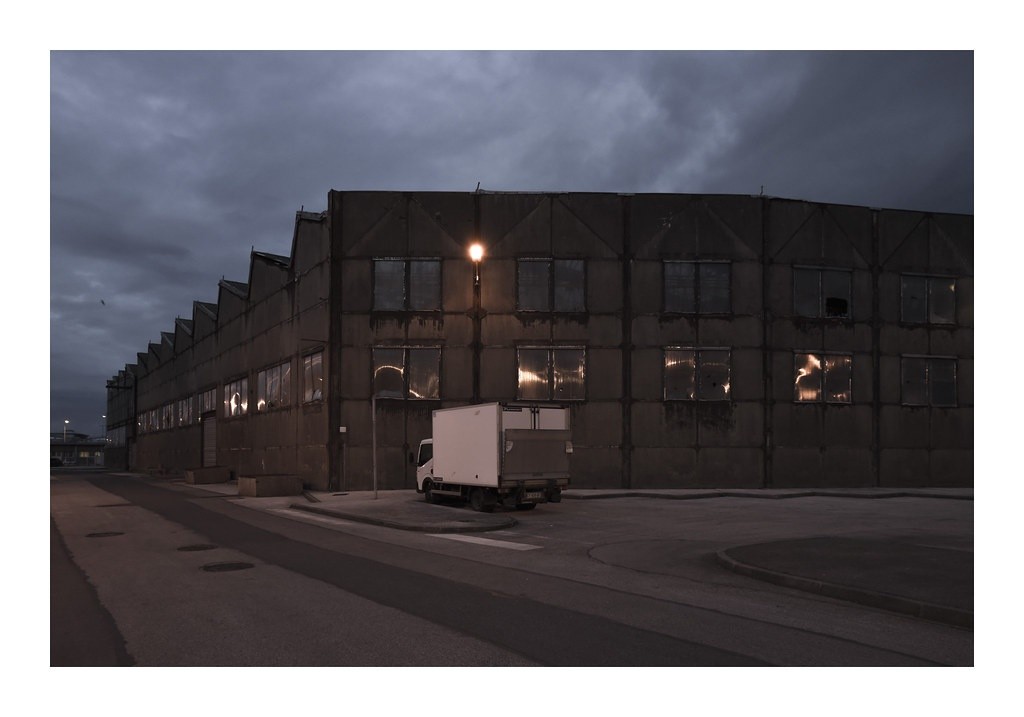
[50,456,63,467]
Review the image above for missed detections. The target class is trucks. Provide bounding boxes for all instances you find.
[407,402,573,514]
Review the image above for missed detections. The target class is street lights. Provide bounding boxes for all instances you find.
[64,420,69,443]
